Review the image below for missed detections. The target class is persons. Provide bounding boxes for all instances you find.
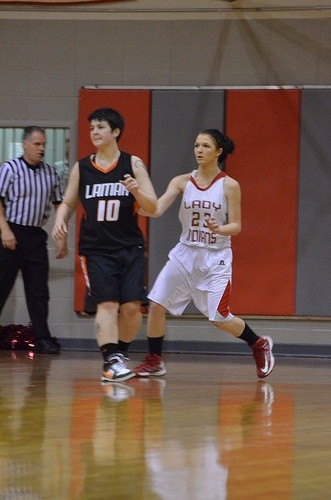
[51,108,158,383]
[0,126,68,353]
[130,129,275,379]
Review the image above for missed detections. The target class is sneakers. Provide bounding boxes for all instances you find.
[100,353,135,382]
[250,335,275,378]
[131,352,166,377]
[33,336,61,354]
